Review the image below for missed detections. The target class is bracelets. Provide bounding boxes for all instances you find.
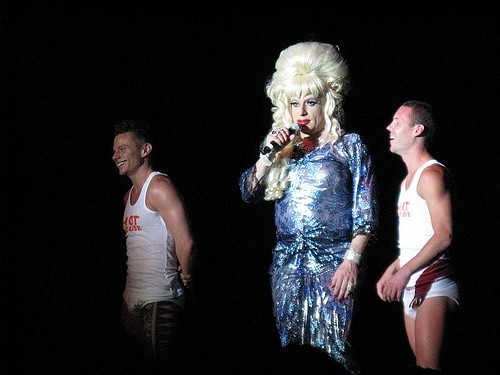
[344,250,362,265]
[260,153,272,166]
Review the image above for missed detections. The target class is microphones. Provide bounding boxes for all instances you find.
[261,122,303,156]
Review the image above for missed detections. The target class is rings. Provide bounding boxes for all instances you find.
[271,130,278,136]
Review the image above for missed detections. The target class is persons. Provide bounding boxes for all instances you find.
[257,341,351,375]
[238,41,377,373]
[377,101,463,371]
[113,119,194,375]
